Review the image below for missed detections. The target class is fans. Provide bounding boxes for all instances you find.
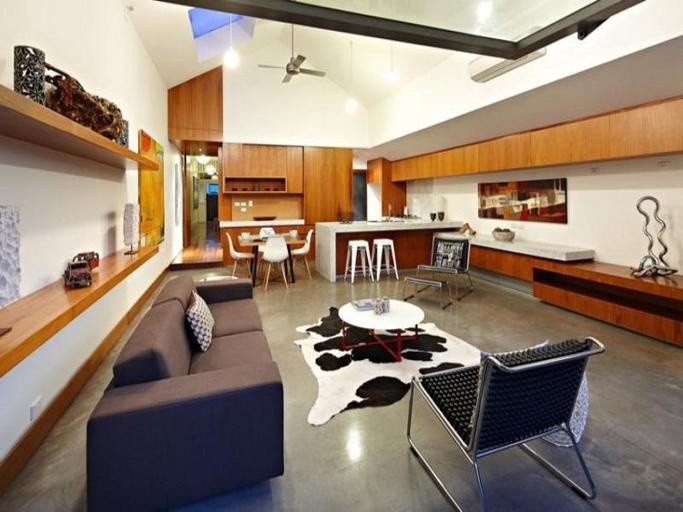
[258,22,325,85]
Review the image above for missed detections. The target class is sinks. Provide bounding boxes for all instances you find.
[367,220,405,222]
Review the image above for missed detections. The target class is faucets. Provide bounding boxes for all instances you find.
[388,203,392,221]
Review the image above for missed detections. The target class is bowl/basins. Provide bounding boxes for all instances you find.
[232,187,279,191]
[492,230,515,241]
[240,231,250,239]
[289,230,297,237]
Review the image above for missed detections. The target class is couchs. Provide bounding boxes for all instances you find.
[85,275,285,510]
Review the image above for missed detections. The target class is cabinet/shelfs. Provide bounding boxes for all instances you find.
[223,175,303,219]
[0,83,157,377]
[217,142,353,267]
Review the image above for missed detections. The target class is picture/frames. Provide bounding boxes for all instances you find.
[477,177,568,224]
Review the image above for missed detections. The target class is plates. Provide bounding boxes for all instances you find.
[254,216,276,221]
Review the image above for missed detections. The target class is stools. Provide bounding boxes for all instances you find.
[343,240,375,284]
[371,238,400,282]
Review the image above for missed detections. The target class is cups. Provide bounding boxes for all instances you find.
[430,211,444,221]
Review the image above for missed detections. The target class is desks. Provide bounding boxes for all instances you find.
[313,219,464,282]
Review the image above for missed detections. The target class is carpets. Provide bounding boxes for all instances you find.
[294,308,486,427]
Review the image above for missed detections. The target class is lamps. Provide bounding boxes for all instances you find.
[197,154,218,180]
[222,13,242,67]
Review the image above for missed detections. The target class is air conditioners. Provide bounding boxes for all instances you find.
[466,26,546,84]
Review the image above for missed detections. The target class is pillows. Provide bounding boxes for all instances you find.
[187,289,216,352]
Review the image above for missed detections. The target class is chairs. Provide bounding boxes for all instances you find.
[224,227,315,289]
[403,233,475,308]
[407,336,606,512]
